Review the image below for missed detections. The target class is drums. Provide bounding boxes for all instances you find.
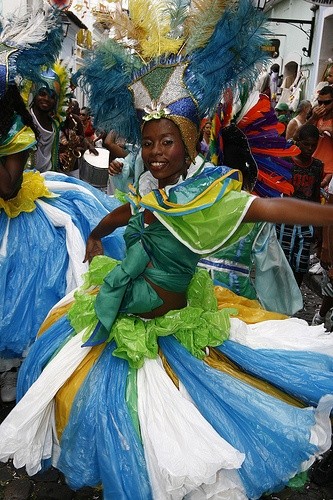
[79,148,109,189]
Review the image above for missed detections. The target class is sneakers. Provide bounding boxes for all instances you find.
[310,253,319,264]
[312,309,326,326]
[0,371,18,402]
[309,262,326,275]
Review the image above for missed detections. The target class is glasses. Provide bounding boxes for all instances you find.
[318,100,333,106]
[80,114,85,116]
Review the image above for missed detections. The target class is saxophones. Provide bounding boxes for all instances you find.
[59,114,81,173]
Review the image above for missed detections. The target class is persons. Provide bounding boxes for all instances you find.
[200,85,333,327]
[0,118,333,500]
[58,100,140,177]
[0,80,126,398]
[10,87,58,172]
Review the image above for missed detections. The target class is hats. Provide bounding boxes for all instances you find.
[32,66,61,97]
[128,59,199,165]
